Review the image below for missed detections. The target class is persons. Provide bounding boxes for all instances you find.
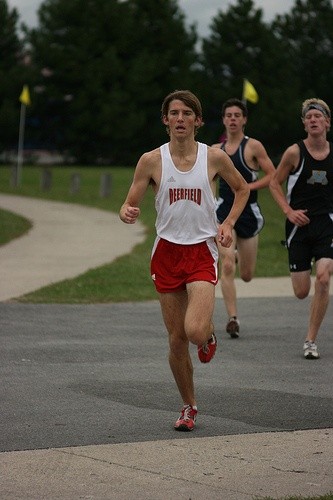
[211,98,276,338]
[270,98,333,358]
[119,89,250,432]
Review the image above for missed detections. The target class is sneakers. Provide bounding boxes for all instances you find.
[174,405,197,431]
[304,340,320,358]
[198,332,218,363]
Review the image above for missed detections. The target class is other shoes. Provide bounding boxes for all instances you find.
[226,320,240,338]
